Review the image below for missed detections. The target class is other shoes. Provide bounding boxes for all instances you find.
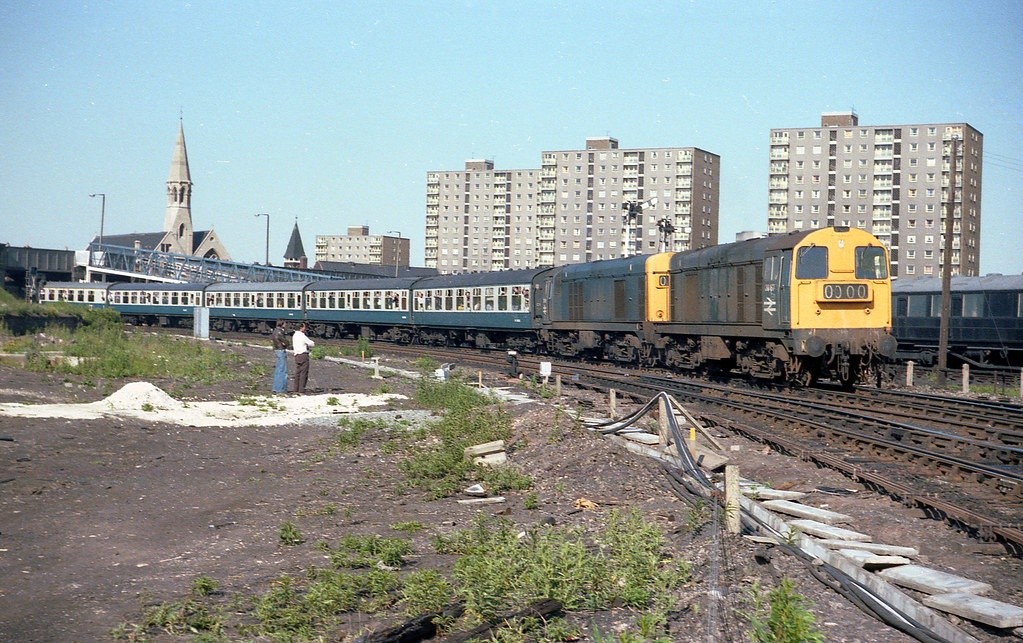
[272,390,307,397]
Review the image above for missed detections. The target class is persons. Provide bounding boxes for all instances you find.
[107,285,530,311]
[292,322,315,395]
[272,319,290,394]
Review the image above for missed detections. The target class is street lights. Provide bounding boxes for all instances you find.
[387,230,401,279]
[254,213,269,266]
[89,193,106,249]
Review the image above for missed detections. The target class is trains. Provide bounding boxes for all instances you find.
[5,225,897,390]
[888,272,1023,369]
